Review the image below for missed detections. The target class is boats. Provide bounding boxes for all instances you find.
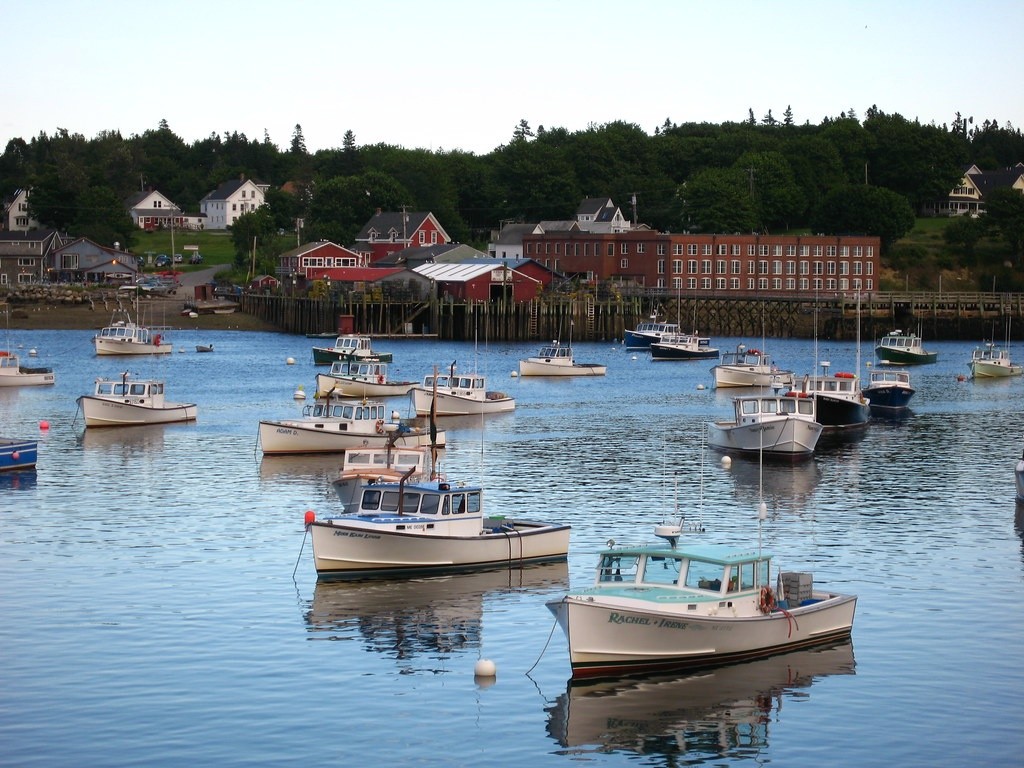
[312,333,392,367]
[624,295,685,350]
[189,311,198,319]
[331,426,427,511]
[0,305,55,387]
[75,367,197,428]
[314,349,421,398]
[795,285,871,430]
[544,421,857,679]
[650,279,719,361]
[0,437,37,472]
[967,316,1023,378]
[708,299,796,388]
[182,309,191,316]
[861,370,915,410]
[406,361,515,417]
[91,270,173,355]
[875,317,937,367]
[305,367,571,578]
[519,340,607,376]
[708,366,824,455]
[259,386,448,456]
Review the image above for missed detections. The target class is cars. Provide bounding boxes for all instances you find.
[155,257,172,268]
[189,254,203,264]
[136,275,179,295]
[173,253,184,263]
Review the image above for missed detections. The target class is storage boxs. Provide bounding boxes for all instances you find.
[778,572,813,604]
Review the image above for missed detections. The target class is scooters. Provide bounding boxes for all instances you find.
[137,256,145,267]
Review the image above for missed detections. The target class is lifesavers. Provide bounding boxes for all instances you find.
[376,420,384,433]
[378,375,384,384]
[761,587,775,613]
[156,334,161,346]
